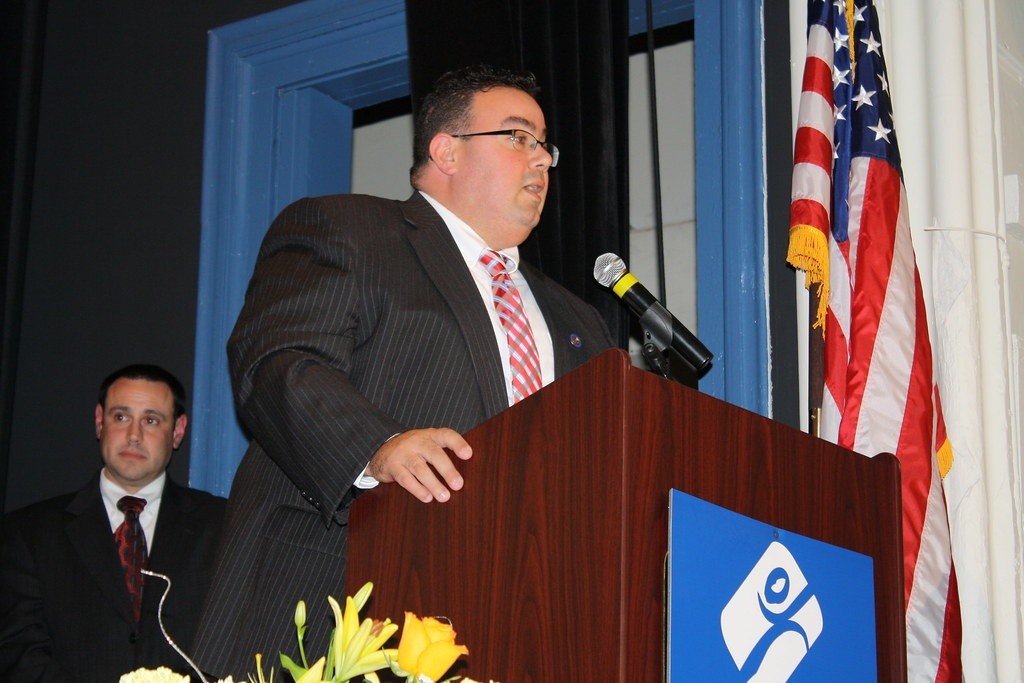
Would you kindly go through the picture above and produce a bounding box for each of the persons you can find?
[183,64,620,683]
[0,364,227,683]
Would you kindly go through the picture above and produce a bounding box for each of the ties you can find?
[111,495,148,626]
[481,250,542,406]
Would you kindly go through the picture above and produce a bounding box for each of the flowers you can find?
[119,581,501,683]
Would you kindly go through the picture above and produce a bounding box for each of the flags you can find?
[786,0,966,683]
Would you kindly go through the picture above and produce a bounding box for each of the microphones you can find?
[594,252,715,371]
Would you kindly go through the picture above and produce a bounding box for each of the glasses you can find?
[428,129,560,168]
